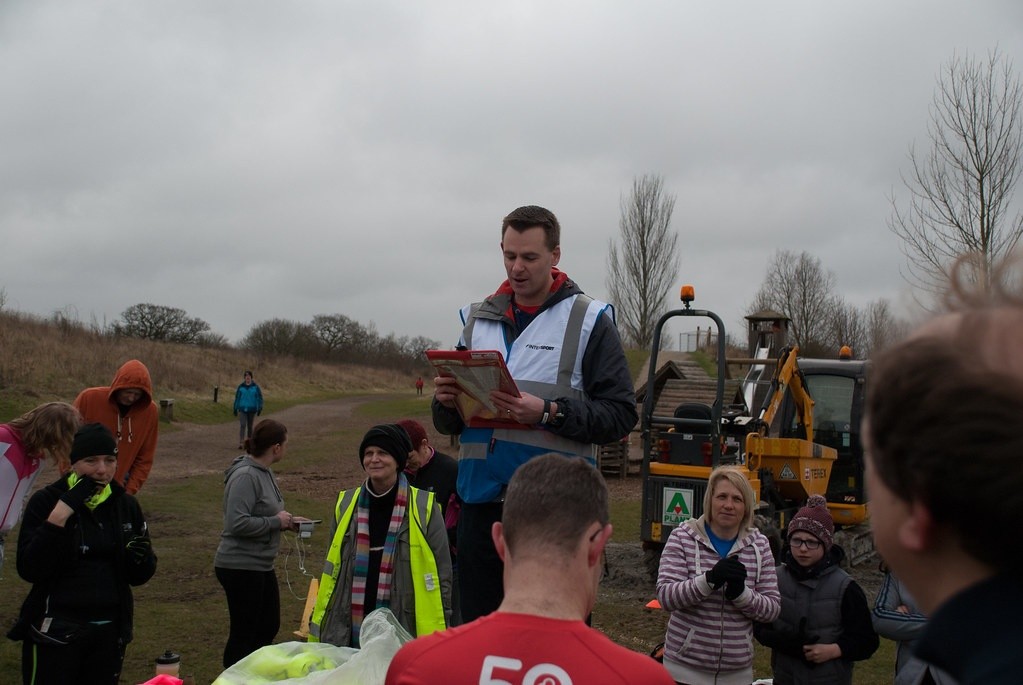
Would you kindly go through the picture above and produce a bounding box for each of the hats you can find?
[360,424,411,474]
[70,423,118,464]
[787,494,834,554]
[243,370,253,379]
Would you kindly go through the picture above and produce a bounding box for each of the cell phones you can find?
[292,519,322,525]
[140,522,147,537]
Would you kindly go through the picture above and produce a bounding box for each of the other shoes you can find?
[238,440,244,449]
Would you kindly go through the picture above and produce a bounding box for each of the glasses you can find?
[789,537,824,549]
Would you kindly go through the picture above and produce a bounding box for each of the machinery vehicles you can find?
[640,284,878,586]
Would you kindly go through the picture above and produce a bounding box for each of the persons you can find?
[6,421,157,685]
[754,496,880,685]
[213,419,311,671]
[432,205,640,628]
[0,402,83,555]
[861,256,1023,685]
[59,359,159,497]
[399,419,462,557]
[655,466,782,685]
[385,454,674,684]
[416,377,424,395]
[307,423,453,652]
[872,570,957,685]
[234,371,264,449]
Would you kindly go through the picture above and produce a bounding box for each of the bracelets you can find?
[536,399,551,426]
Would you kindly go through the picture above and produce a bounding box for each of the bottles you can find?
[155,649,180,680]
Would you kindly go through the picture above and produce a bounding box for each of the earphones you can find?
[299,562,304,570]
[302,567,306,574]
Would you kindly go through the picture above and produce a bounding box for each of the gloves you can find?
[125,527,154,567]
[59,474,105,512]
[706,555,747,601]
[770,617,821,661]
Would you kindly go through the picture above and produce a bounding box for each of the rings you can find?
[508,409,510,415]
